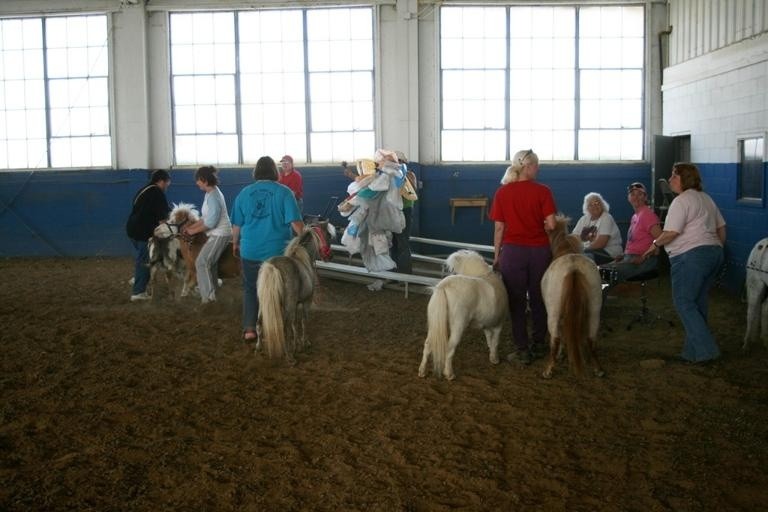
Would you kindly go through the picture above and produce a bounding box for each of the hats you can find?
[396,149,408,165]
[627,181,647,194]
[280,155,293,165]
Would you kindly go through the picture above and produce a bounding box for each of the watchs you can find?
[652,238,661,249]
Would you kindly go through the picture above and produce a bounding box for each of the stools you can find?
[598,260,618,316]
[626,246,664,330]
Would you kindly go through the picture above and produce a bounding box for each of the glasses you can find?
[522,149,533,161]
[626,184,648,192]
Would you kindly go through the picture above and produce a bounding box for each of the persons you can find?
[343,150,418,275]
[490,148,555,365]
[277,154,307,215]
[125,169,169,302]
[641,161,727,365]
[229,155,306,343]
[601,183,663,287]
[185,164,233,307]
[571,191,623,266]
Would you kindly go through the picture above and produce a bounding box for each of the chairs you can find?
[658,179,675,226]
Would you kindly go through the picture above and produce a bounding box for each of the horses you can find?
[741,236,768,350]
[256,218,333,367]
[540,212,609,379]
[154,201,243,301]
[417,249,509,381]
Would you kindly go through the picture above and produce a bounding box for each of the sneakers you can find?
[690,355,723,366]
[244,329,257,342]
[507,348,531,365]
[532,343,549,358]
[671,353,691,365]
[128,277,136,287]
[130,291,151,303]
